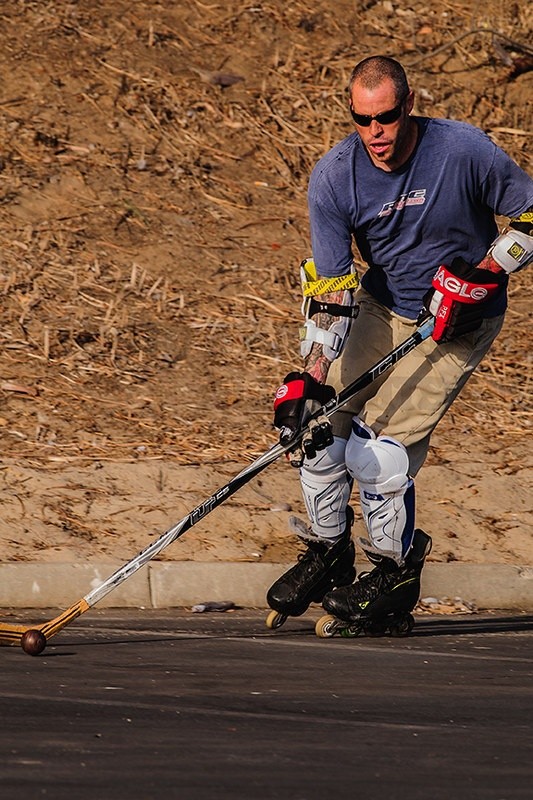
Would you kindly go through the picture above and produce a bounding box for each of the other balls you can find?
[21,629,47,655]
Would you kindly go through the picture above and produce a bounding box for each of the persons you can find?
[259,56,533,639]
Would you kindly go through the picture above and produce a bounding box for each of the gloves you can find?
[271,371,337,469]
[415,258,507,344]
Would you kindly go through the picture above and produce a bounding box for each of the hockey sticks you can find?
[0,311,436,646]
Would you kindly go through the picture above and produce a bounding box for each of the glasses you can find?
[349,101,402,128]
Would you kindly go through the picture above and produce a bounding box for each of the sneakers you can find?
[264,505,358,632]
[314,528,434,638]
